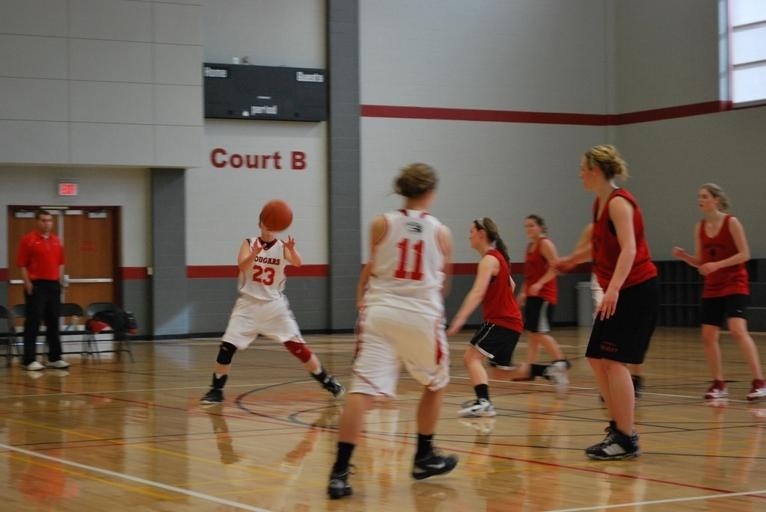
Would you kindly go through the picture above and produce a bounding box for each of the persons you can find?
[199,214,344,404]
[673,183,766,400]
[518,215,563,365]
[446,218,568,417]
[578,224,644,402]
[16,209,71,371]
[551,144,659,461]
[327,163,459,499]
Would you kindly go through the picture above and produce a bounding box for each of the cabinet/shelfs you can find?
[653,260,766,332]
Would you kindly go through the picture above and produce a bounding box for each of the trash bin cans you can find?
[574,281,593,328]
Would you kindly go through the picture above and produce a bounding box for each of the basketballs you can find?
[261,201,293,232]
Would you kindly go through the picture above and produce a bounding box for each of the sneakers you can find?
[322,375,345,397]
[17,361,45,371]
[545,359,571,397]
[585,420,639,461]
[47,361,70,369]
[200,389,224,404]
[329,479,352,498]
[412,453,458,480]
[746,377,766,400]
[704,380,727,398]
[457,395,495,418]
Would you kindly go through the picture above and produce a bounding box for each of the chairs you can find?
[0,303,138,368]
